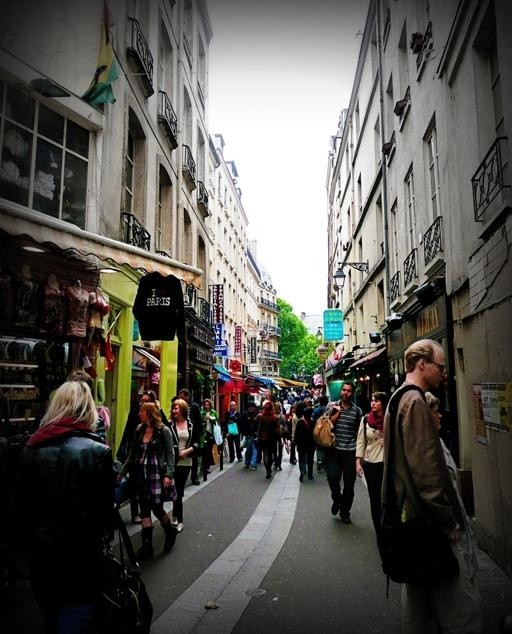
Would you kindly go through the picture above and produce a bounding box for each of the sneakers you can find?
[191,476,200,488]
[328,498,352,525]
[171,513,191,533]
[225,454,323,482]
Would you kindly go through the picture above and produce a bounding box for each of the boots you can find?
[159,519,176,554]
[134,526,154,562]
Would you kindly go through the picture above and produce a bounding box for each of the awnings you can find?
[249,375,309,389]
[350,346,386,369]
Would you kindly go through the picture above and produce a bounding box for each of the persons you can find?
[0,380,154,633]
[117,389,219,554]
[312,381,388,563]
[220,386,315,482]
[383,339,484,634]
[14,266,111,336]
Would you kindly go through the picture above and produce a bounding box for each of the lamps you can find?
[333,259,370,289]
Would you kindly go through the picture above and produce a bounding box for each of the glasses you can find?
[425,360,447,374]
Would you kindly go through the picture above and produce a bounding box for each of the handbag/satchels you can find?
[86,553,157,633]
[377,504,461,586]
